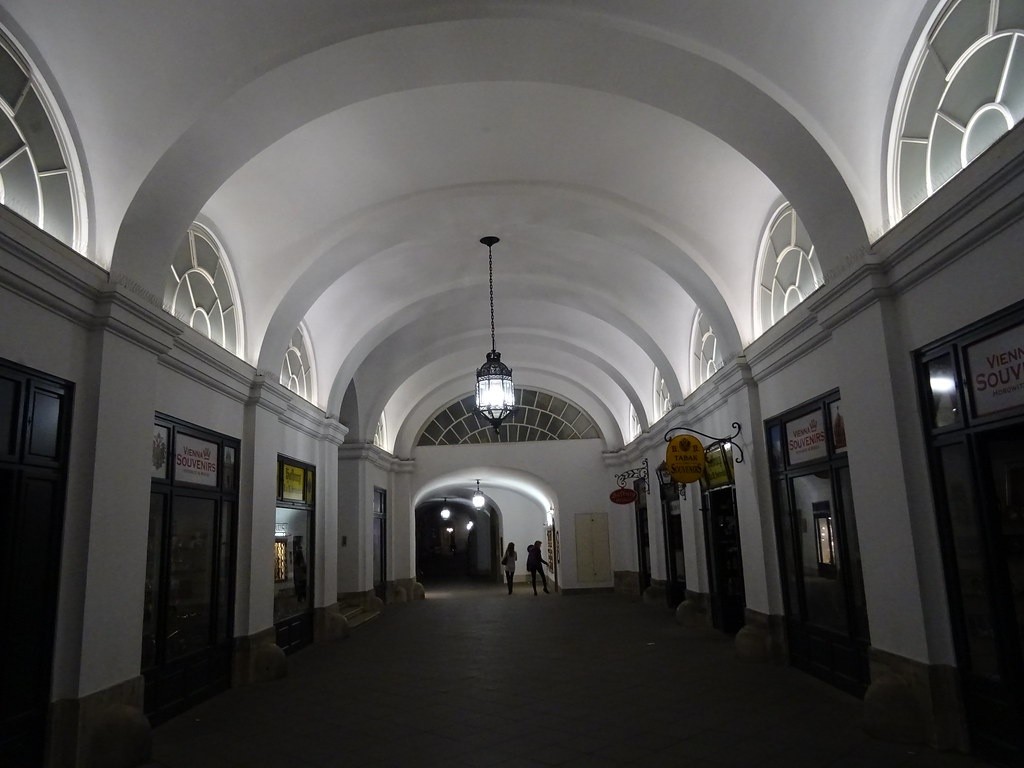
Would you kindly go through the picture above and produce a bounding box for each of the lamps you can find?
[440,496,451,520]
[475,236,520,437]
[471,479,486,510]
[655,460,687,502]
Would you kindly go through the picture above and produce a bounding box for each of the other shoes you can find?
[543,588,549,594]
[534,592,537,595]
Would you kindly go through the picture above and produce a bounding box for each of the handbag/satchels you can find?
[502,551,508,565]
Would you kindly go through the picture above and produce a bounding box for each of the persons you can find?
[502,541,517,595]
[527,541,550,596]
